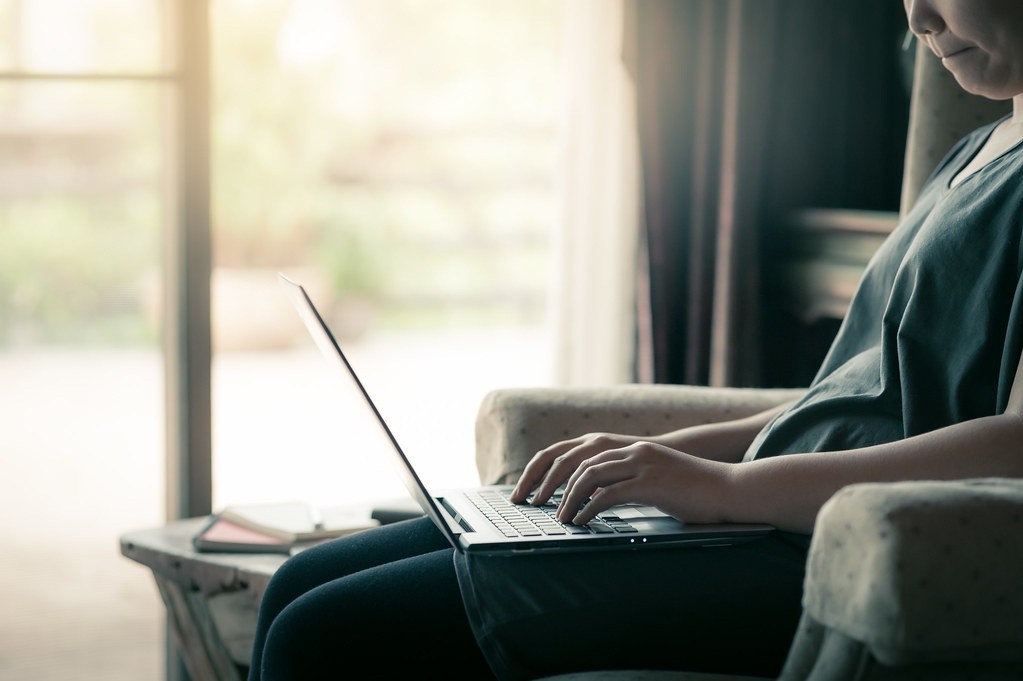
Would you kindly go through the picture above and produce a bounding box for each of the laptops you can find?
[273,272,778,556]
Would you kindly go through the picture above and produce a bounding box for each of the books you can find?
[193,498,381,554]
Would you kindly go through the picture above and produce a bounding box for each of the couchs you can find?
[475,383,1023,681]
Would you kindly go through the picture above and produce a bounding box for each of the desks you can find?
[121,514,358,680]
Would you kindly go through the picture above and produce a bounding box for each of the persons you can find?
[253,0,1023,681]
